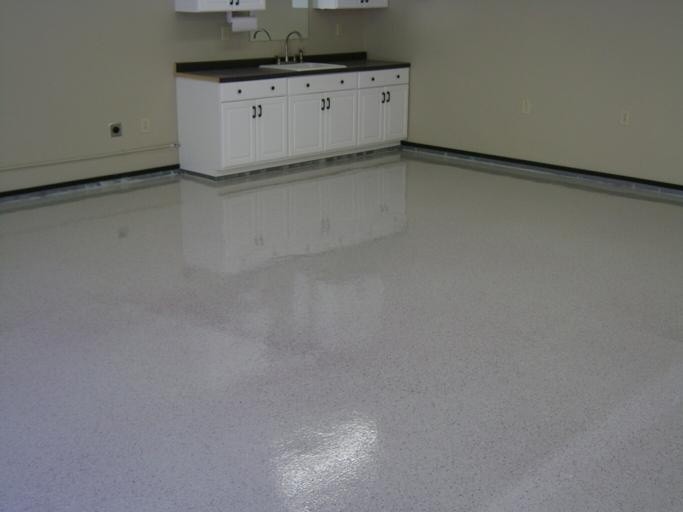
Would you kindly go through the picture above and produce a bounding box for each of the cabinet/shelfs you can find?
[175,77,288,181]
[358,66,410,153]
[313,0,390,10]
[292,0,308,8]
[288,71,358,166]
[174,0,267,13]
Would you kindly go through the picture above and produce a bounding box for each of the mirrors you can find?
[249,0,310,41]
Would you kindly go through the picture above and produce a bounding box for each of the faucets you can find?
[254,29,271,41]
[285,31,302,63]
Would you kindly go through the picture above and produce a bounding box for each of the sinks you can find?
[275,63,338,71]
